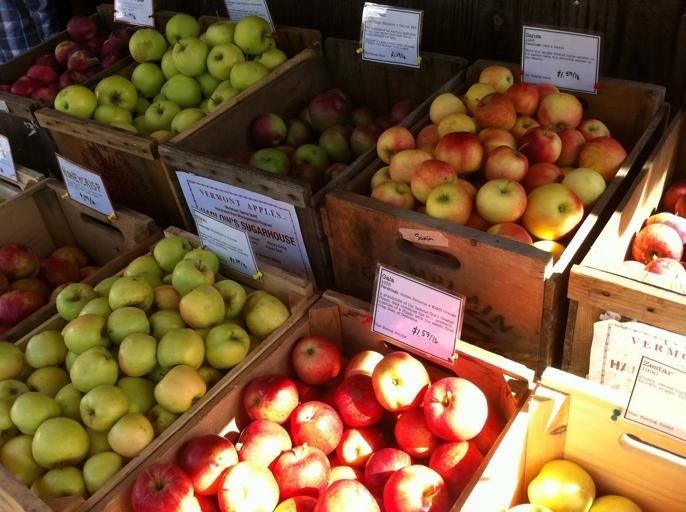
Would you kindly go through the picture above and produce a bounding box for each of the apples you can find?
[0,16,131,104]
[0,237,292,500]
[54,10,289,140]
[507,457,643,512]
[622,177,686,280]
[370,65,627,263]
[128,334,508,512]
[2,240,100,333]
[221,88,422,183]
